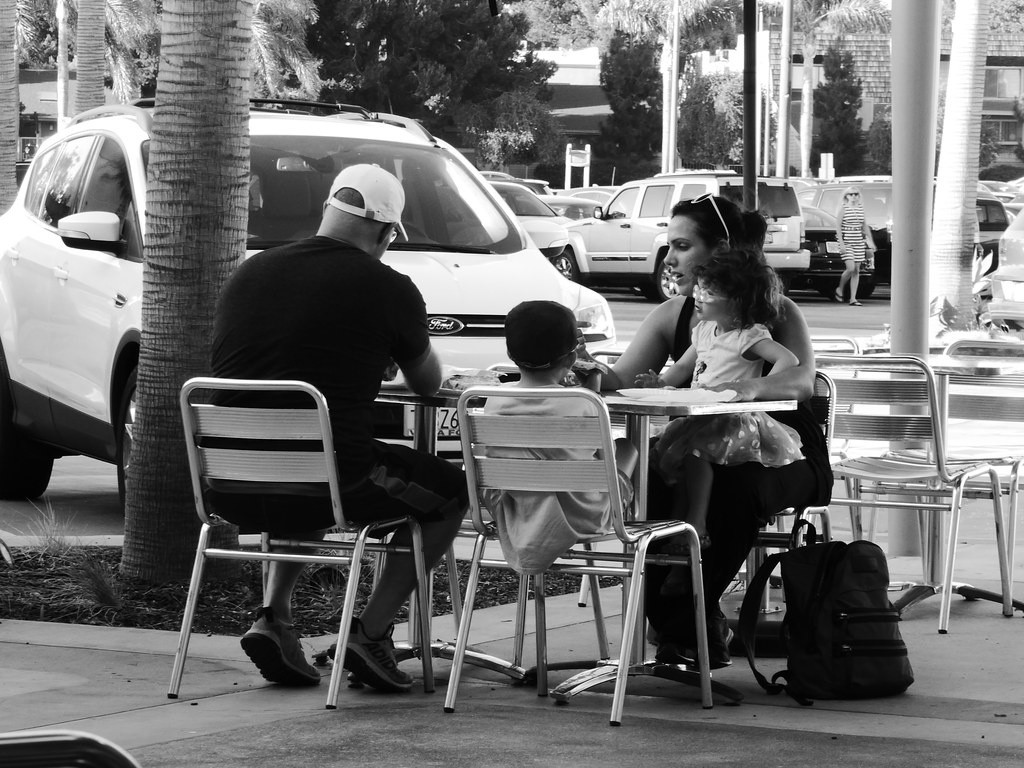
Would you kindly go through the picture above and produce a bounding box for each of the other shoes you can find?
[659,534,711,555]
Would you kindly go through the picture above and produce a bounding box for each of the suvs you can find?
[474,169,1024,330]
[0,98,617,522]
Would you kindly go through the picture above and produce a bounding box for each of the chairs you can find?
[165,337,1024,725]
[256,170,323,239]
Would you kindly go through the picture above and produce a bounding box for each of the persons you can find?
[476,299,640,571]
[195,164,469,693]
[576,193,834,667]
[832,187,876,304]
[632,248,800,554]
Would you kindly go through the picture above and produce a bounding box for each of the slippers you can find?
[849,301,863,306]
[834,286,843,302]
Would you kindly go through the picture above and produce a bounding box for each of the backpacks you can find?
[738,520,916,705]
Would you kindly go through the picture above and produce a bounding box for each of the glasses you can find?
[692,192,732,240]
[692,284,726,303]
[846,193,859,196]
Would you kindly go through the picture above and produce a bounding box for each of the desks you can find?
[374,382,802,706]
[820,351,1024,621]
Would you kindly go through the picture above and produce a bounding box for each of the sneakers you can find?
[655,624,734,667]
[240,606,322,687]
[329,617,414,694]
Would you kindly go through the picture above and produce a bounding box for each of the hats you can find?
[326,164,409,243]
